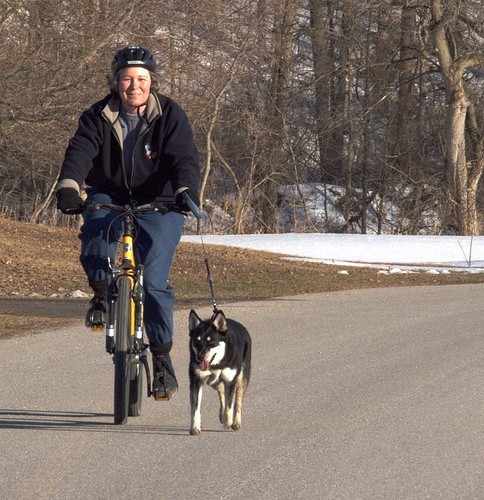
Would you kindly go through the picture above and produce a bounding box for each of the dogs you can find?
[189,309,251,435]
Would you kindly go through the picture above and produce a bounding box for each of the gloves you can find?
[172,186,200,212]
[55,179,84,214]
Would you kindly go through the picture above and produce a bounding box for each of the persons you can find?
[56,47,200,398]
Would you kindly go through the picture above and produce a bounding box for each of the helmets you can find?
[111,45,156,77]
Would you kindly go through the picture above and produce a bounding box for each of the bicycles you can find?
[61,198,188,427]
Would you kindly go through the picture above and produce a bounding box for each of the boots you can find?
[149,340,179,398]
[85,278,108,327]
